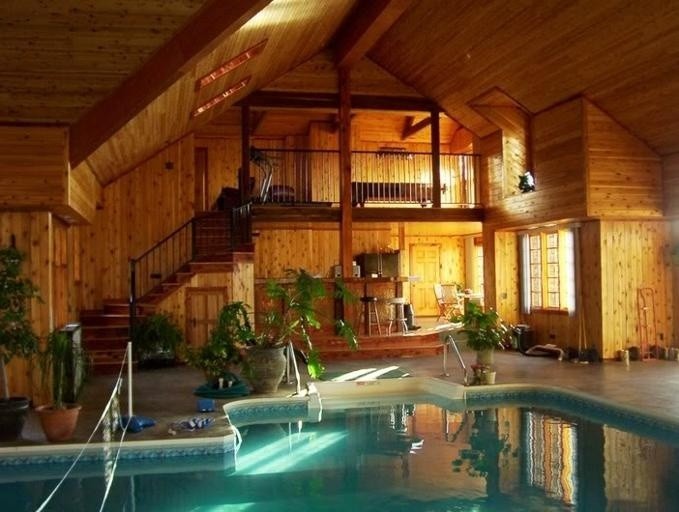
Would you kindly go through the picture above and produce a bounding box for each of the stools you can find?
[356,296,382,338]
[385,297,410,337]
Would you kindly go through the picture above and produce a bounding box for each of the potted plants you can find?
[1,245,47,443]
[195,303,251,401]
[237,271,358,397]
[472,364,496,385]
[33,326,90,440]
[449,301,509,366]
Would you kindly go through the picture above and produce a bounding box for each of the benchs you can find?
[352,182,430,208]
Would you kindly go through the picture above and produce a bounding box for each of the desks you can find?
[456,293,484,316]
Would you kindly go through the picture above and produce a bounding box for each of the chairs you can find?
[432,283,460,322]
